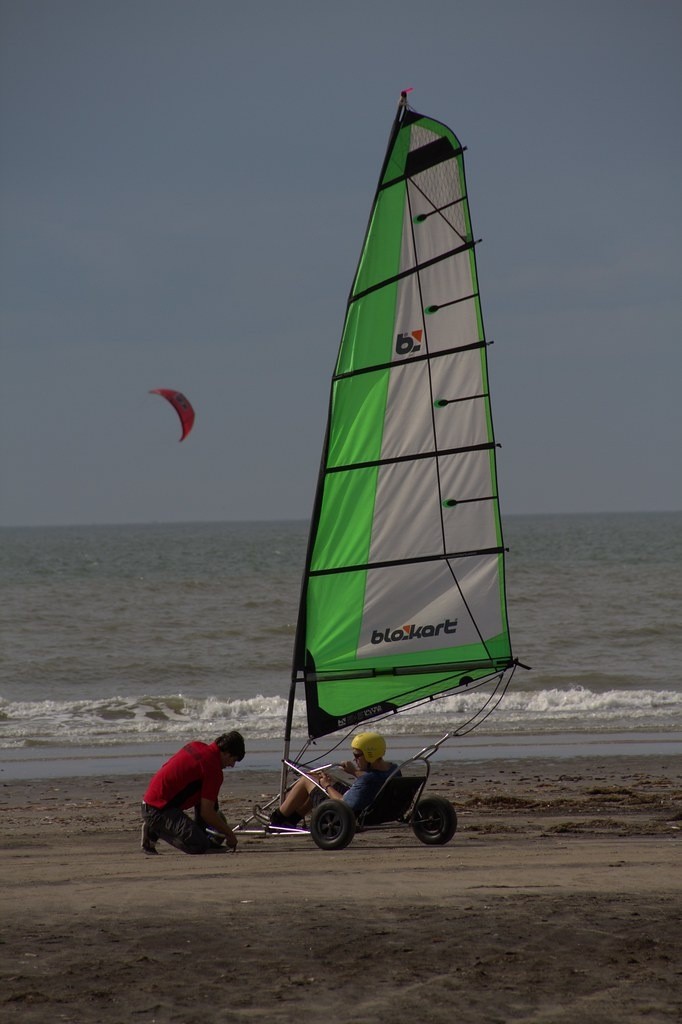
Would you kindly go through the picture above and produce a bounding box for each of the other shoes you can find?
[140,821,160,856]
[264,822,297,833]
[253,804,288,833]
[212,846,227,853]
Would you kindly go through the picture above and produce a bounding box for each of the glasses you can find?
[352,752,363,759]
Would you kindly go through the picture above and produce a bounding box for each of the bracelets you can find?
[325,785,330,794]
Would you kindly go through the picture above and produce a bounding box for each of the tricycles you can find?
[224,760,526,847]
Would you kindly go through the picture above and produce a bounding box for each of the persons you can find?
[140,730,246,855]
[254,732,402,833]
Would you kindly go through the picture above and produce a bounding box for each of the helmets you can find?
[351,731,386,762]
